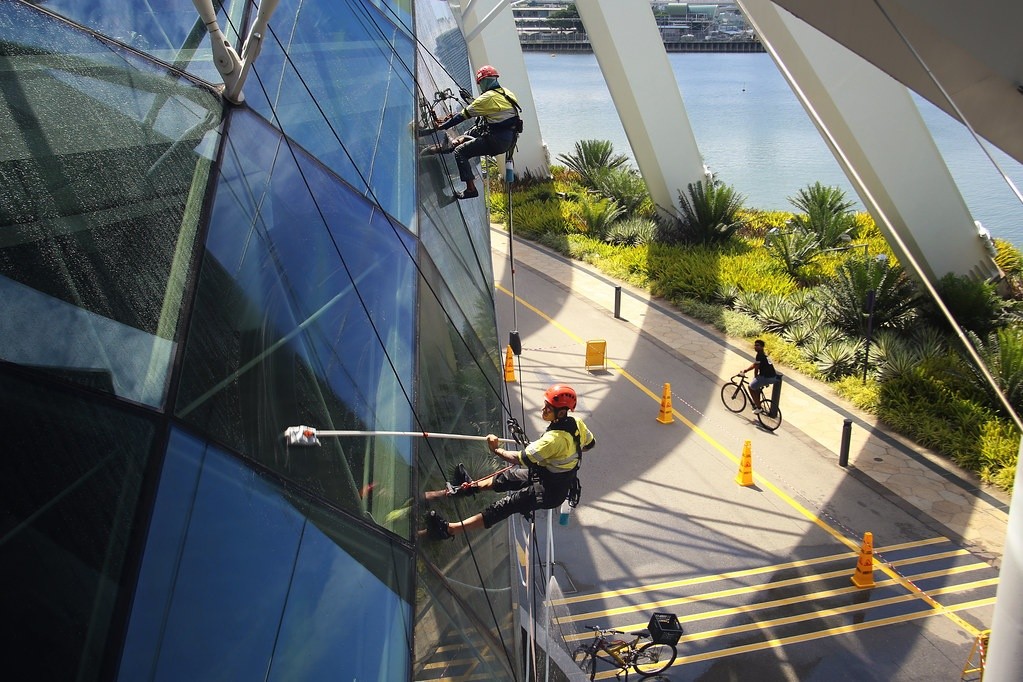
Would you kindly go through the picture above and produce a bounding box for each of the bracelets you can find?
[743,369,747,373]
[494,446,500,454]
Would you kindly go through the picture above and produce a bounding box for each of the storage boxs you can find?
[647,612,684,646]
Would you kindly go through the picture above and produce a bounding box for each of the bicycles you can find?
[720,370,782,432]
[571,624,678,682]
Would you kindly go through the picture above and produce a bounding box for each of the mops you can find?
[282,425,530,447]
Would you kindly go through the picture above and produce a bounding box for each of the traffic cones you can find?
[850,532,878,588]
[655,380,677,425]
[502,343,516,383]
[736,440,757,487]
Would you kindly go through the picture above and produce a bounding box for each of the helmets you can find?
[477,65,500,84]
[543,384,577,412]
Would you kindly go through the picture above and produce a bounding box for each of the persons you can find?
[434,64,523,200]
[740,340,778,414]
[427,384,597,542]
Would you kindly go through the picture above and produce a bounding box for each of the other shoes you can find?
[430,510,456,542]
[446,131,457,151]
[752,408,762,414]
[458,463,475,497]
[456,190,479,199]
